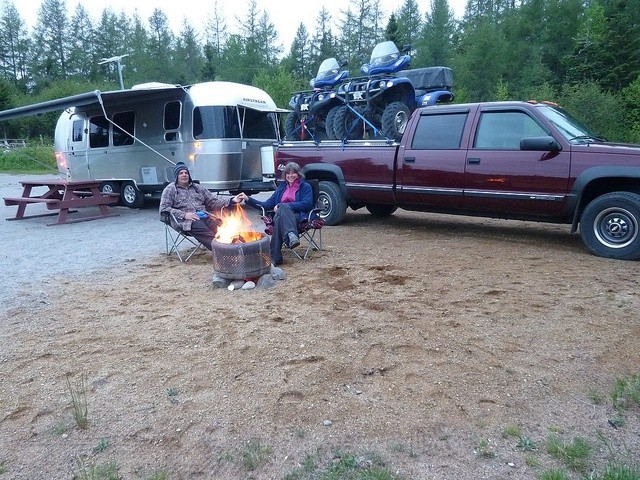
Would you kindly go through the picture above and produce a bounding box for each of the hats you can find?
[173,160,198,194]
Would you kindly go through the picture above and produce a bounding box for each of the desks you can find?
[5,181,120,227]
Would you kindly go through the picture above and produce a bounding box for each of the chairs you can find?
[256,179,322,259]
[162,180,205,261]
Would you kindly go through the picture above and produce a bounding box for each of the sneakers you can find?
[272,258,283,266]
[288,232,300,249]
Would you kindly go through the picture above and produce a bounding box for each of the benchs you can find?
[61,189,121,214]
[3,195,62,226]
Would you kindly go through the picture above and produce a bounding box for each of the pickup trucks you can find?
[275,100,640,259]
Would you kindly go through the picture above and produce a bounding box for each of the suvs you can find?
[333,41,454,140]
[286,57,349,141]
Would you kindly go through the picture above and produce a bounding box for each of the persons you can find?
[237,162,316,267]
[159,161,243,252]
